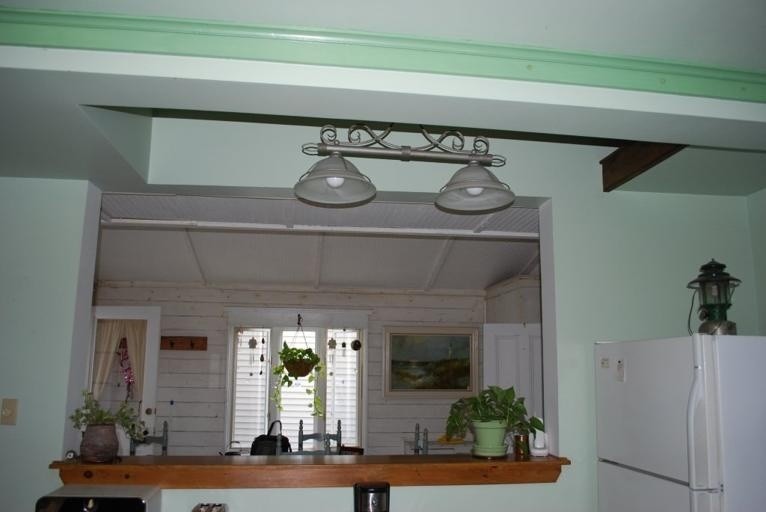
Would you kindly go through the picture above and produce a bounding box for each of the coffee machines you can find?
[352,481,389,512]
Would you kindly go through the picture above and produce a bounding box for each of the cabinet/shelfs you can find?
[482,323,545,456]
[403,437,479,457]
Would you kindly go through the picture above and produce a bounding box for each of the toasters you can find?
[191,502,229,512]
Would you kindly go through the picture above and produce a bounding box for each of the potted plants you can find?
[444,385,545,457]
[67,390,147,465]
[268,340,325,420]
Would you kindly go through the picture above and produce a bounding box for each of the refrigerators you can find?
[592,337,765,512]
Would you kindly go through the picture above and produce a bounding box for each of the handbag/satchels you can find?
[252,435,288,455]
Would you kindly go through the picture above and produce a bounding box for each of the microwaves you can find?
[34,483,161,512]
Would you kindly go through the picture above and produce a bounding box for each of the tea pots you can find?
[217,440,242,456]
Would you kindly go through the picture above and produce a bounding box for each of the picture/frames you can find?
[382,325,480,401]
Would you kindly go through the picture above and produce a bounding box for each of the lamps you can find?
[292,121,515,212]
[684,257,743,336]
